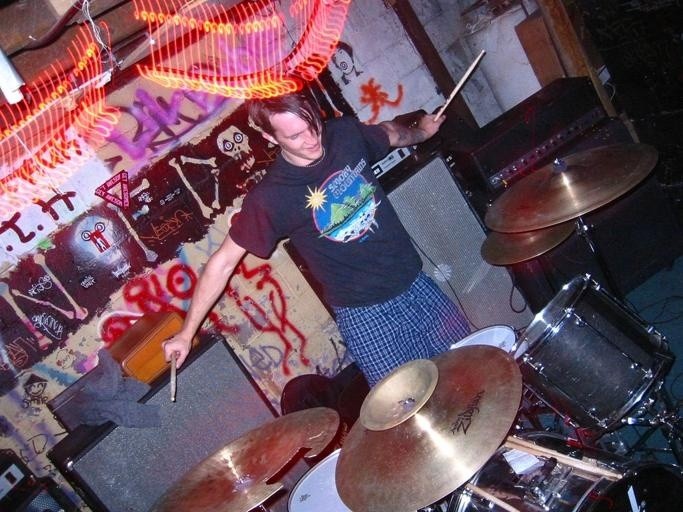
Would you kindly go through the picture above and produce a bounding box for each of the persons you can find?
[161,85,471,391]
[330,41,363,85]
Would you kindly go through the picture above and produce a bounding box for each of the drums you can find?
[450,432,681,511]
[285,448,350,511]
[510,276,670,436]
[450,326,517,353]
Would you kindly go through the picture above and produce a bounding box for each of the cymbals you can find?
[484,144,658,232]
[147,406,339,512]
[335,346,522,511]
[481,221,576,265]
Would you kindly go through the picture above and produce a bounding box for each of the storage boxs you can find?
[46,368,143,432]
[108,310,202,387]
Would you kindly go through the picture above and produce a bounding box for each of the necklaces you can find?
[281,145,326,168]
